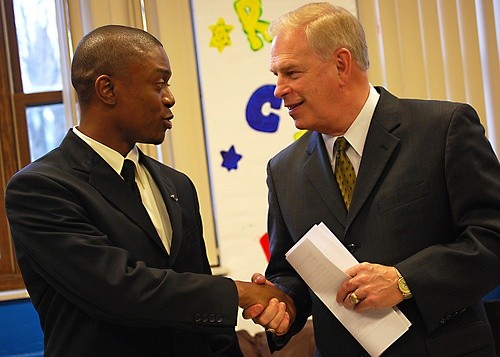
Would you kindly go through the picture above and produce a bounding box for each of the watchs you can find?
[396,268,413,299]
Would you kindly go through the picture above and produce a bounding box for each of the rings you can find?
[266,328,276,333]
[350,292,360,305]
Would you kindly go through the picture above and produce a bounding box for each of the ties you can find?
[121,159,142,202]
[334,137,356,209]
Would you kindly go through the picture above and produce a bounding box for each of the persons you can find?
[242,2,500,357]
[5,25,296,357]
